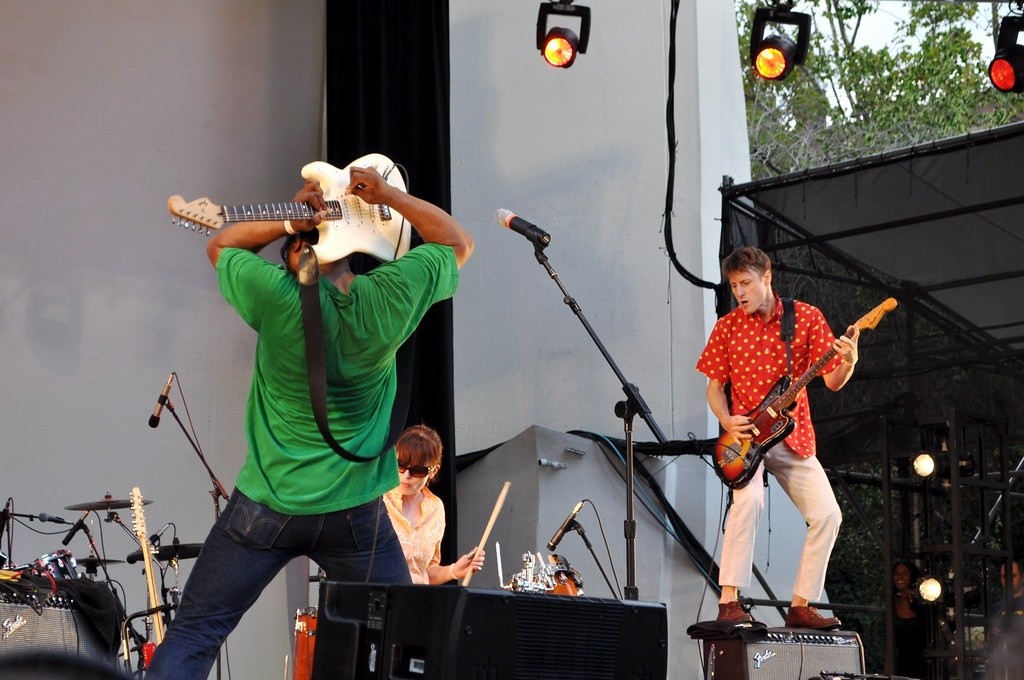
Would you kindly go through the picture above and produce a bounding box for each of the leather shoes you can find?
[785,605,842,630]
[716,601,752,623]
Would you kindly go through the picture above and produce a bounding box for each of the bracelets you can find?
[283,219,297,235]
[449,563,459,580]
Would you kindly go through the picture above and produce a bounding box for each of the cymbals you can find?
[75,556,125,566]
[64,499,154,511]
[129,542,204,561]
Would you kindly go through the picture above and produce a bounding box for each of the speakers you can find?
[702,626,866,680]
[311,582,668,680]
[0,590,117,670]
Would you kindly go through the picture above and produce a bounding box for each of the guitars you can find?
[167,153,412,266]
[129,487,166,670]
[293,605,318,680]
[712,298,899,490]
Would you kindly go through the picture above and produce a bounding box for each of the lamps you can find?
[913,572,982,609]
[750,0,811,82]
[988,0,1024,93]
[910,446,973,480]
[536,0,591,69]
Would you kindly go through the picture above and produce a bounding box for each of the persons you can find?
[146,166,473,680]
[694,247,859,631]
[382,425,485,585]
[990,558,1024,680]
[891,559,930,680]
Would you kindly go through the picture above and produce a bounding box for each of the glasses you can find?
[397,462,436,478]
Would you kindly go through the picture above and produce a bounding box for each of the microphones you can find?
[808,676,853,680]
[62,511,90,546]
[0,498,11,537]
[149,373,174,428]
[547,500,585,552]
[493,209,550,244]
[39,513,73,525]
[127,523,171,564]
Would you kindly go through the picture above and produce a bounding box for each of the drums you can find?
[28,549,81,579]
[510,565,580,596]
